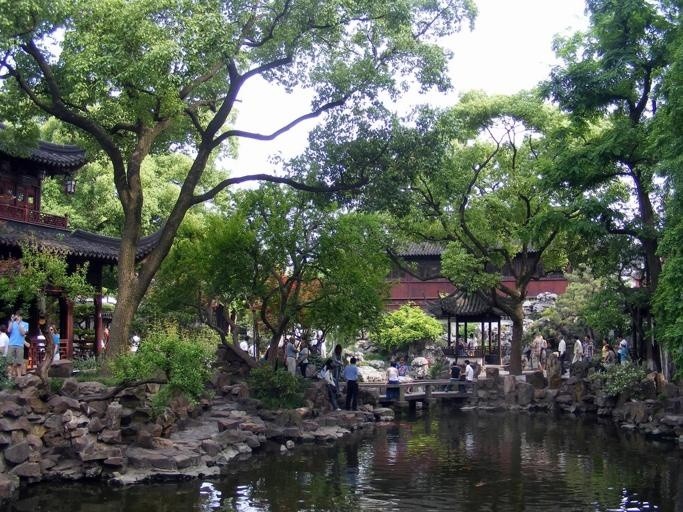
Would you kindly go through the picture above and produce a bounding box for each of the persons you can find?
[5,310,30,382]
[0,309,60,363]
[129,331,139,352]
[466,332,477,356]
[283,334,360,412]
[526,329,630,377]
[248,341,256,360]
[443,362,462,391]
[384,362,399,400]
[457,360,473,393]
[456,334,466,353]
[239,335,248,353]
[396,356,413,393]
